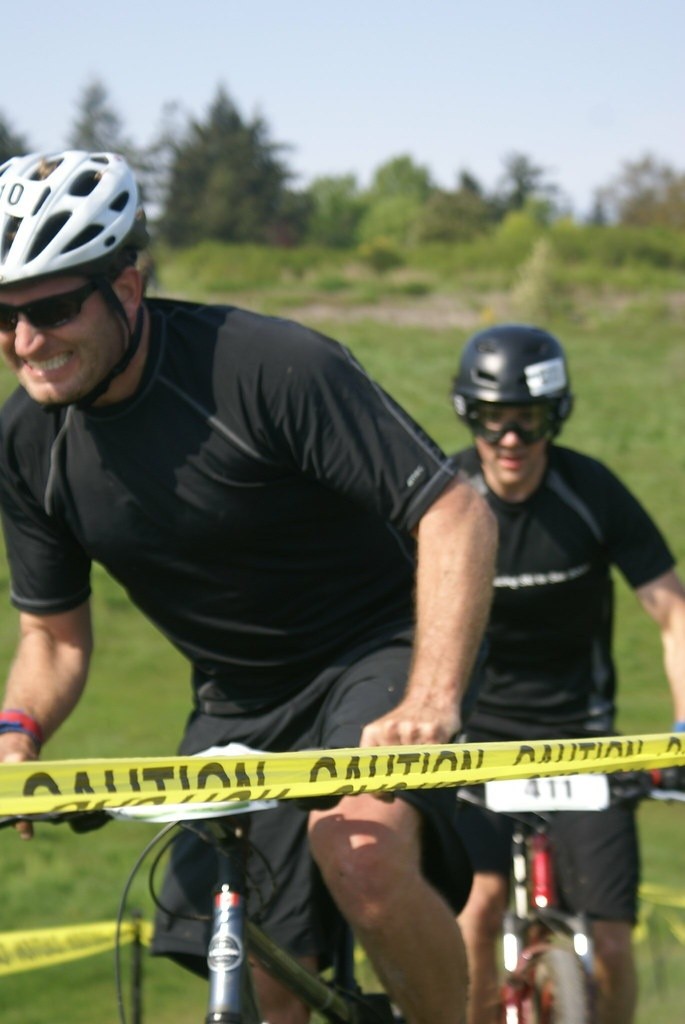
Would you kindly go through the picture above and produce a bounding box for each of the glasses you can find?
[0,256,137,332]
[471,404,554,445]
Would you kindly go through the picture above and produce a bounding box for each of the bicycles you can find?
[455,764,684,1023]
[1,740,404,1024]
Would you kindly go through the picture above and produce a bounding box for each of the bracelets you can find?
[672,722,685,732]
[0,709,43,756]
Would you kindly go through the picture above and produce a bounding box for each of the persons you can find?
[0,148,498,1024]
[446,325,685,1024]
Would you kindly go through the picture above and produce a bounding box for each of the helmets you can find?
[0,148,151,291]
[451,325,586,428]
